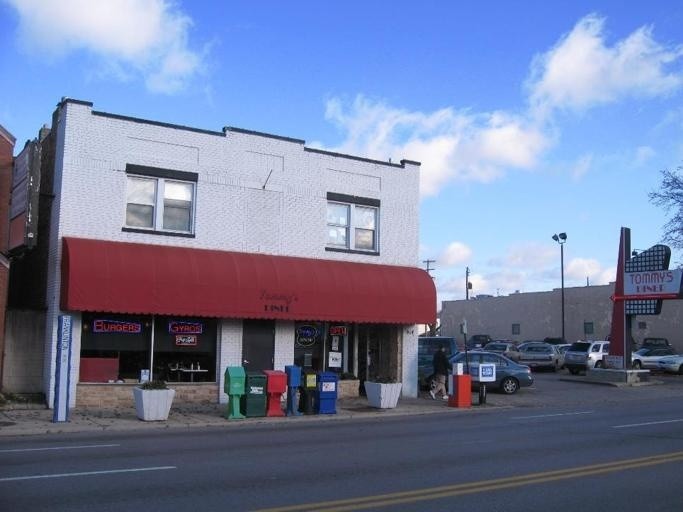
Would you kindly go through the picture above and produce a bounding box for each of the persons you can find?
[429,344,453,400]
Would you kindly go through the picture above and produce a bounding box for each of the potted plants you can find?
[133,380,175,422]
[336,370,403,409]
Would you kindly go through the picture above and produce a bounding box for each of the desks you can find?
[169,368,208,382]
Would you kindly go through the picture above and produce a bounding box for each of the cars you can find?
[418,336,683,394]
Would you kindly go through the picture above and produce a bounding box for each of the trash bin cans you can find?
[447,374,472,408]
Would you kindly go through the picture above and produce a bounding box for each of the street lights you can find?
[553,233,566,339]
